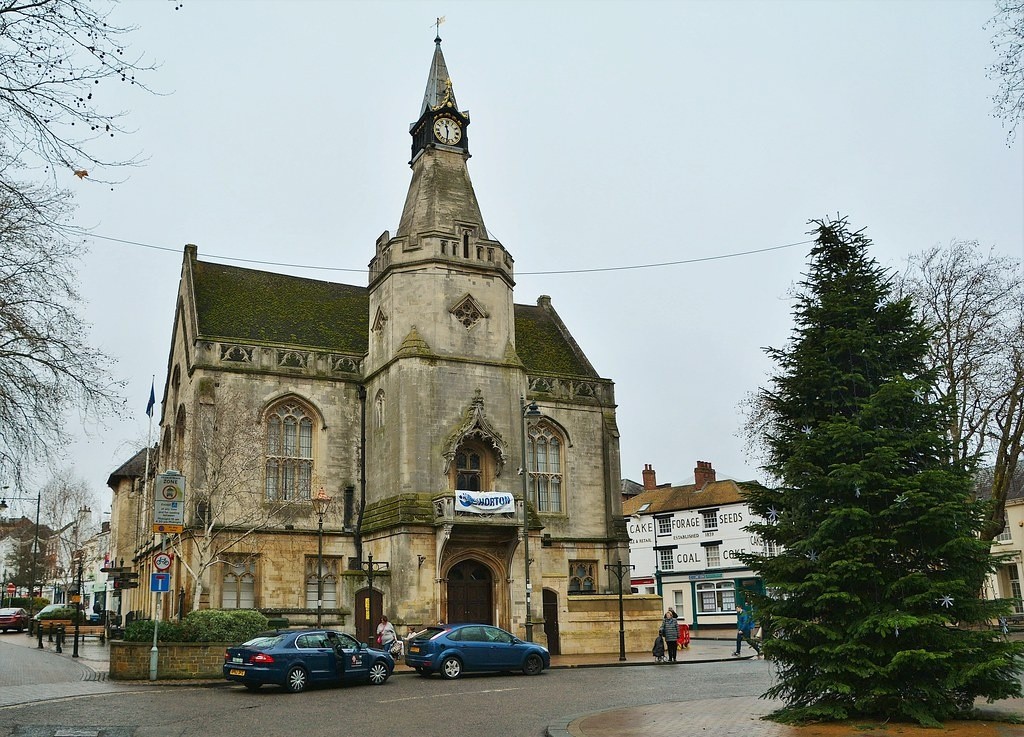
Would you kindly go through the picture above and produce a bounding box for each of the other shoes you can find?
[674,660,676,661]
[734,651,740,655]
[668,658,672,661]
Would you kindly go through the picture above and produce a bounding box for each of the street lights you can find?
[0,490,40,606]
[518,392,545,644]
[312,487,332,629]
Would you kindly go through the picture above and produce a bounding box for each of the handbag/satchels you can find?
[388,641,404,661]
[377,635,382,644]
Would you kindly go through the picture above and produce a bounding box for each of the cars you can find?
[222,628,396,694]
[402,622,551,681]
[33,603,85,622]
[0,607,31,633]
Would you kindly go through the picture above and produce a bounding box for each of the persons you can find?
[93,601,115,624]
[403,627,416,641]
[659,607,678,661]
[732,604,763,656]
[377,615,397,652]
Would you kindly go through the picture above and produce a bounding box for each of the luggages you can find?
[652,636,668,662]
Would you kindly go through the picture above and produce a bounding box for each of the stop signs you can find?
[7,584,15,593]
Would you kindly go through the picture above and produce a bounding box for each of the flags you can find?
[146,382,155,418]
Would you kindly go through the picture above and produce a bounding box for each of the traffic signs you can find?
[100,567,139,589]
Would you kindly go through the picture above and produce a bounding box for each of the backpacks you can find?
[748,614,755,629]
